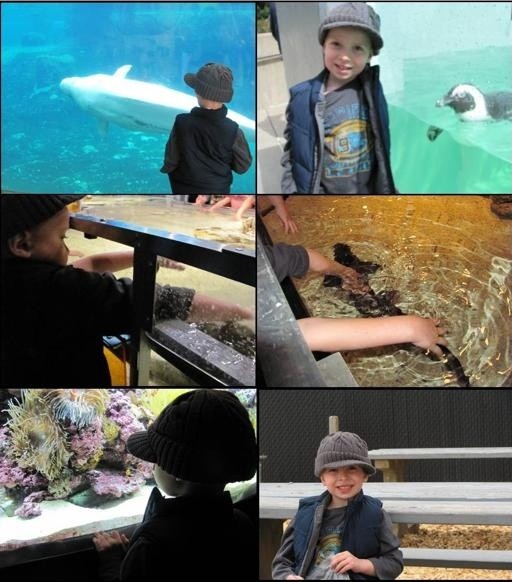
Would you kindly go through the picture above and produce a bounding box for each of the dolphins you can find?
[60,64,255,143]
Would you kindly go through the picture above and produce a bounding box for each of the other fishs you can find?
[323,243,470,387]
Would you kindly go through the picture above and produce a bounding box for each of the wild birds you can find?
[434,83,512,122]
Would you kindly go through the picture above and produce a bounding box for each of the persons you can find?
[91,389,260,581]
[161,62,252,195]
[269,431,403,582]
[201,195,255,221]
[1,193,256,388]
[192,195,209,208]
[279,2,401,194]
[264,241,448,360]
[260,193,301,234]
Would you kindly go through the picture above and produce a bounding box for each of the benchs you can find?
[259,447,512,580]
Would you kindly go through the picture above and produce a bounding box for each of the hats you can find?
[0,194,85,242]
[318,2,383,50]
[128,390,257,483]
[314,432,375,476]
[184,63,233,102]
